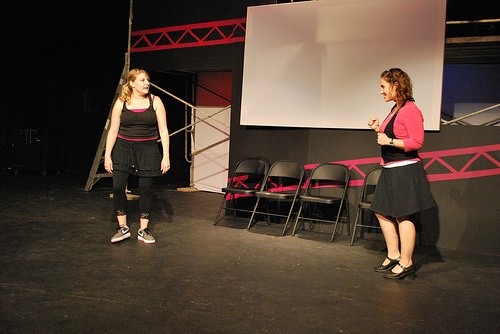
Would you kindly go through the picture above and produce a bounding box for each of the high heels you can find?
[373,256,416,282]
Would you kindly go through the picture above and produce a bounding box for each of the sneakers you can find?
[111,226,156,244]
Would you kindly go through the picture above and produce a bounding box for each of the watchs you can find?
[389,137,395,148]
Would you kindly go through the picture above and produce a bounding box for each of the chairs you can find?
[247,161,304,236]
[350,167,383,246]
[292,163,350,242]
[214,157,268,231]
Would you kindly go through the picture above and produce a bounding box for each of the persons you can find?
[368,67,439,280]
[104,68,169,244]
